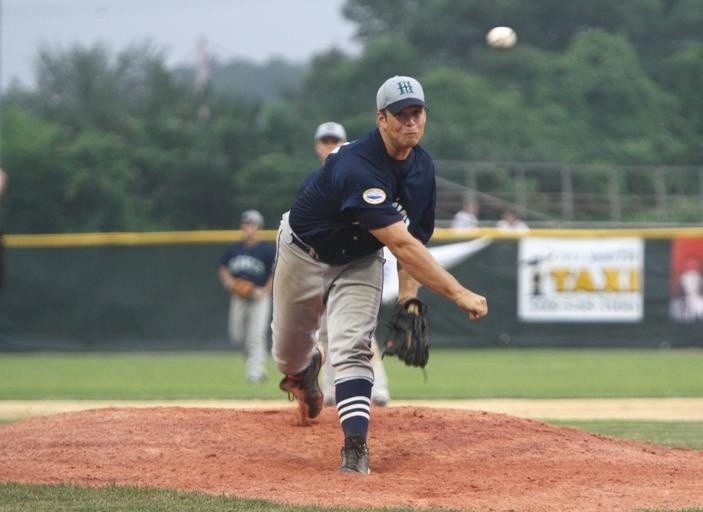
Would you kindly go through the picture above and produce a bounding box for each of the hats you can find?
[376,75,430,115]
[243,210,264,228]
[316,122,345,140]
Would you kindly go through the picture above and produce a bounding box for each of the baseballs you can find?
[485,26,516,48]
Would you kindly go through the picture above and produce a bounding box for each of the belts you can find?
[289,233,325,262]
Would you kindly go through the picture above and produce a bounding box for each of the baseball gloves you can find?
[382,295,431,368]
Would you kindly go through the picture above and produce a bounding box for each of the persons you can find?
[314,120,389,407]
[270,75,488,475]
[498,210,530,232]
[453,197,479,229]
[218,209,277,385]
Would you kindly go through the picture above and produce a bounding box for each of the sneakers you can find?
[336,433,370,476]
[372,389,388,405]
[323,386,337,405]
[280,341,326,418]
